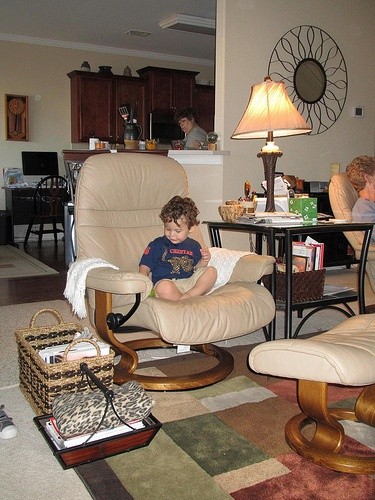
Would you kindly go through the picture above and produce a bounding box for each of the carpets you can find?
[72,329,375,500]
[0,244,59,278]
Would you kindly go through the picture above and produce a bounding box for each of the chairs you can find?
[72,153,276,390]
[328,172,374,292]
[23,175,70,255]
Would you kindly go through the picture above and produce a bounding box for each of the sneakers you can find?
[0,404,17,439]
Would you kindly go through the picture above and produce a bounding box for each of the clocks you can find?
[3,94,28,141]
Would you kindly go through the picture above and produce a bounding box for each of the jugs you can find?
[122,122,143,139]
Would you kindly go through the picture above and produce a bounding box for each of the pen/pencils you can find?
[245,181,257,201]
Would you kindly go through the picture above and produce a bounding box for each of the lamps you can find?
[230,75,312,222]
[159,14,216,37]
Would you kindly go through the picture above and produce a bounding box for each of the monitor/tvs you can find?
[21,151,58,183]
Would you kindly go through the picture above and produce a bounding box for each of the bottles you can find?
[139,139,146,150]
[174,140,185,151]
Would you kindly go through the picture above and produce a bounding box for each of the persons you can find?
[139,196,217,301]
[179,112,208,150]
[348,156,375,242]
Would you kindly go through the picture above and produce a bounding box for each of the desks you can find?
[201,220,374,339]
[308,192,335,220]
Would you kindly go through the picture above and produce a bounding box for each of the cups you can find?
[225,199,240,206]
[239,201,256,216]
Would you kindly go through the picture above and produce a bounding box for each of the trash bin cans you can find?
[0,210,11,245]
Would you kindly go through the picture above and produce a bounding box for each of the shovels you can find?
[119,106,129,122]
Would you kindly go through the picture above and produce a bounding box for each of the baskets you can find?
[263,268,327,303]
[15,309,162,470]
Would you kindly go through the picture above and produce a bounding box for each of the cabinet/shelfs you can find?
[3,187,72,249]
[67,65,215,144]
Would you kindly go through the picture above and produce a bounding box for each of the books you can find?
[39,341,111,364]
[45,418,145,448]
[292,241,324,272]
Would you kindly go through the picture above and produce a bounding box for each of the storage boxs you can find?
[289,197,318,223]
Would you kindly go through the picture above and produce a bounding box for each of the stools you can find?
[248,314,375,474]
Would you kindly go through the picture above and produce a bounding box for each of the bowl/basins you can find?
[218,205,244,222]
[86,137,139,150]
